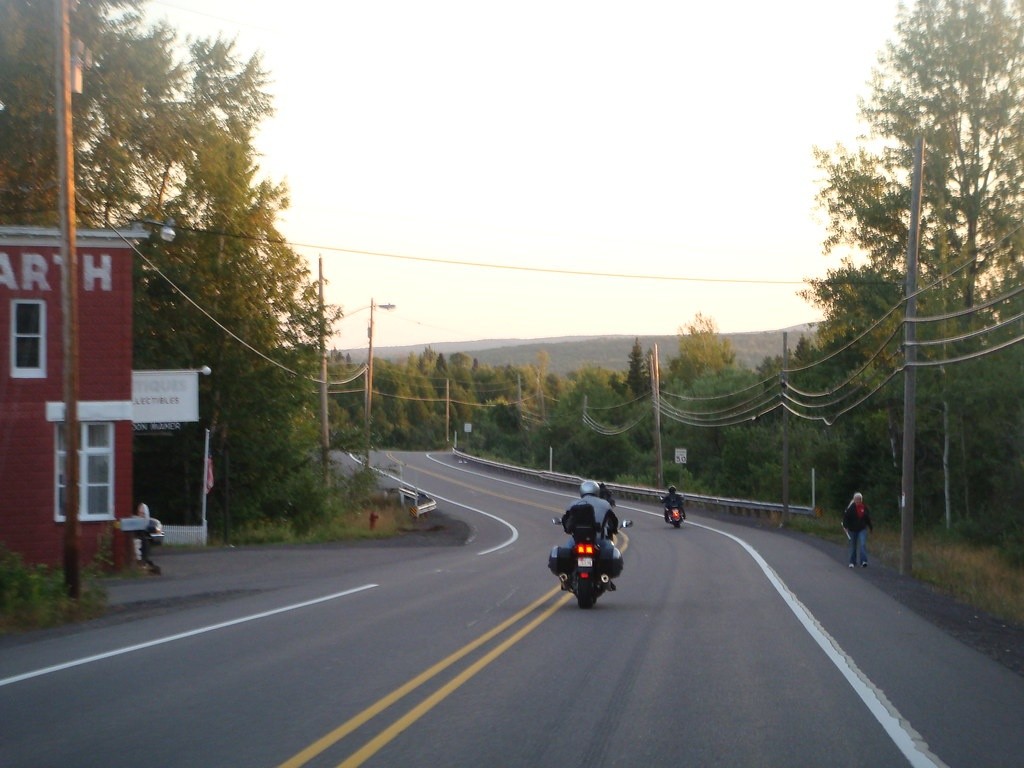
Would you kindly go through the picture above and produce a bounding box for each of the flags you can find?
[206,434,214,494]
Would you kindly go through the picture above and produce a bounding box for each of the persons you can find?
[842,492,874,568]
[562,479,619,549]
[660,485,687,524]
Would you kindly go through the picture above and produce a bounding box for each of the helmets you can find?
[579,481,600,498]
[669,486,676,493]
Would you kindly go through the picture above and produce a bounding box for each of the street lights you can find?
[364,297,396,471]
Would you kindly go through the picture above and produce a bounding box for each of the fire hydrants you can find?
[369,511,379,531]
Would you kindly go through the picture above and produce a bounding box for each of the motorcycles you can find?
[548,518,634,609]
[659,493,688,528]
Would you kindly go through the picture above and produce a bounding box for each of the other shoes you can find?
[610,581,616,591]
[861,562,868,568]
[849,563,854,568]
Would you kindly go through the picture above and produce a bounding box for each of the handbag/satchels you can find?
[567,500,595,533]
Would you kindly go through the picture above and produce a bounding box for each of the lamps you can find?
[199,365,212,375]
[159,225,176,243]
